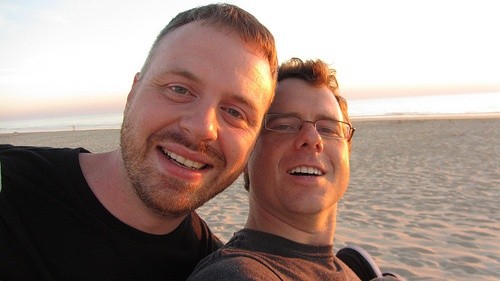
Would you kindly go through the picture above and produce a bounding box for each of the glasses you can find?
[265,112,356,142]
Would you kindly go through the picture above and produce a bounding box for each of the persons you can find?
[187,58,361,281]
[1,3,278,281]
